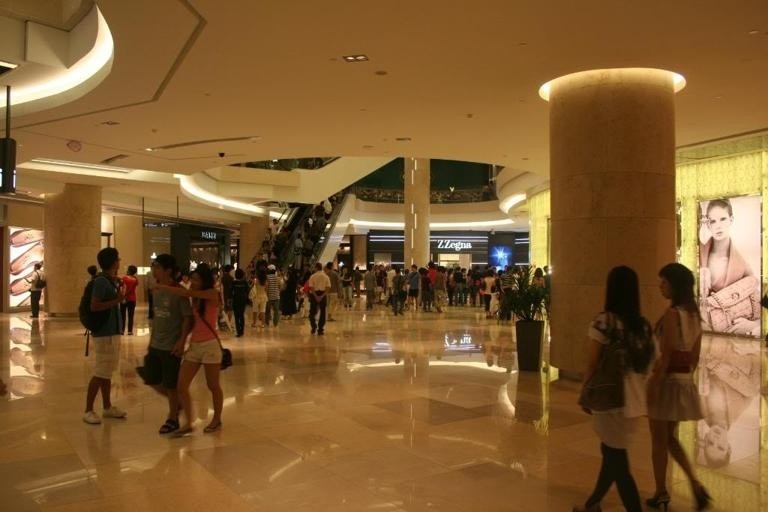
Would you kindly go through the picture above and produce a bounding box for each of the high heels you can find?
[646,492,669,512]
[694,487,710,511]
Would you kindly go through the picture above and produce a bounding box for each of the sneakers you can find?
[574,503,602,512]
[82,407,221,438]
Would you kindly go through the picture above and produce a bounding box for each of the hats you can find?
[267,265,274,270]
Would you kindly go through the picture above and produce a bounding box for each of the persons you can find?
[22,317,49,373]
[646,263,715,512]
[88,259,339,337]
[700,201,761,339]
[571,265,655,512]
[143,437,196,489]
[156,264,223,437]
[697,334,761,471]
[83,247,128,424]
[24,263,45,317]
[252,196,333,276]
[113,257,124,334]
[136,254,193,434]
[190,309,550,383]
[80,424,120,484]
[338,260,551,324]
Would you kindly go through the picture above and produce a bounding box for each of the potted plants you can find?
[495,265,550,370]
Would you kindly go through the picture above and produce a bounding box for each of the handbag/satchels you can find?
[248,287,257,302]
[36,280,45,289]
[580,353,623,412]
[705,276,760,332]
[220,349,231,369]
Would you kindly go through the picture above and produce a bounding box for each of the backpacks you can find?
[79,275,118,330]
